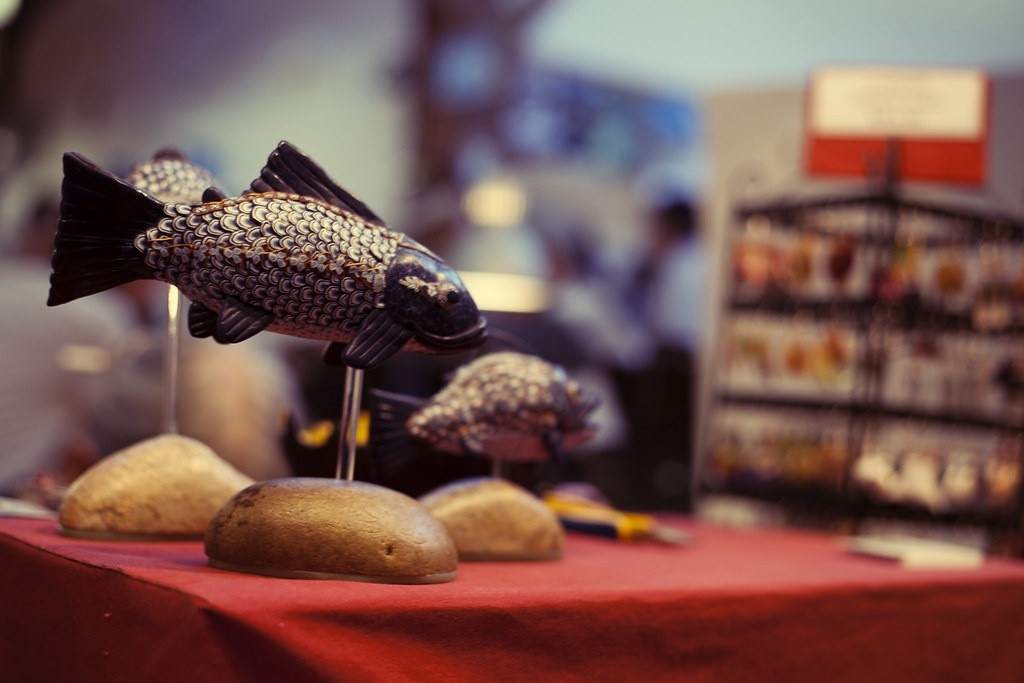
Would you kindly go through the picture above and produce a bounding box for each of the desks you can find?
[0,502,1024,681]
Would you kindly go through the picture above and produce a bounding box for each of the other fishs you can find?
[117,146,230,211]
[369,350,602,468]
[47,141,490,369]
